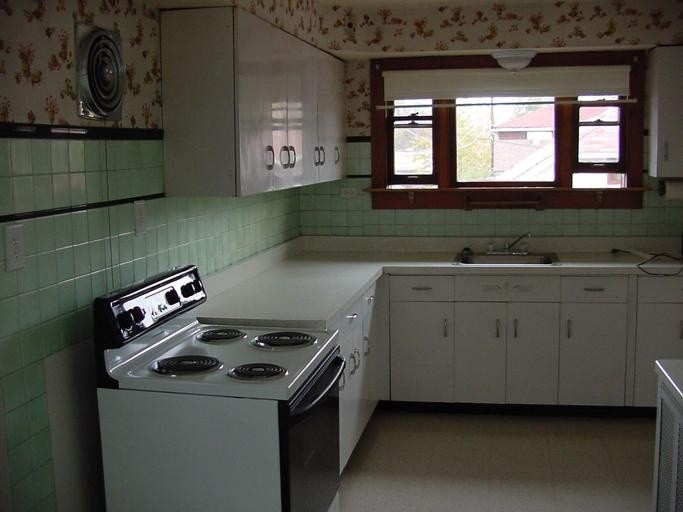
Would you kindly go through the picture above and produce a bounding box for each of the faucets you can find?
[503,231,532,250]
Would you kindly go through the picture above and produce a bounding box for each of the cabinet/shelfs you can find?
[648,44,683,179]
[634,275,683,406]
[389,275,454,403]
[559,275,628,407]
[161,6,346,199]
[337,295,381,477]
[454,274,559,406]
[361,276,385,434]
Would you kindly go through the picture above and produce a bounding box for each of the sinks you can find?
[460,254,554,264]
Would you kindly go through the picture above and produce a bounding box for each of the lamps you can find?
[490,50,538,71]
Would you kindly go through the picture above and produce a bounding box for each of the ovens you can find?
[96,346,348,511]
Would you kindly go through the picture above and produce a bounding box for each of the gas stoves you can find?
[106,317,339,400]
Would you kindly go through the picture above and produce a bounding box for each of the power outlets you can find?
[134,200,146,236]
[5,223,25,272]
